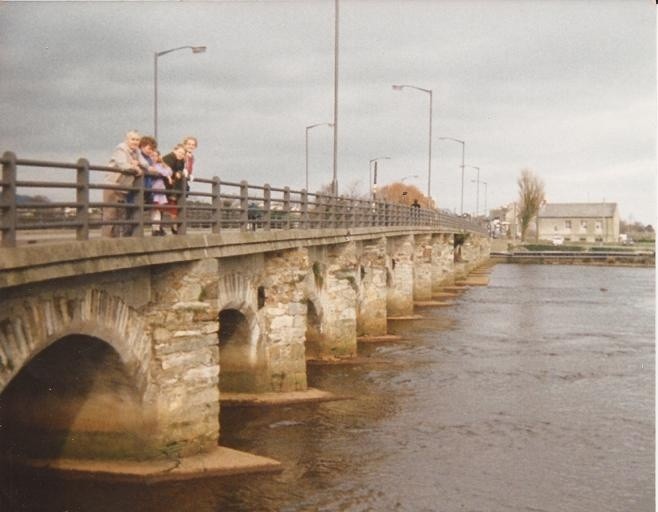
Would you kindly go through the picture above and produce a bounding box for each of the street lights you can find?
[437,136,465,216]
[305,122,334,190]
[458,165,480,213]
[368,157,390,200]
[154,42,207,141]
[392,84,433,206]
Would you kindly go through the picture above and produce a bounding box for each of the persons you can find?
[100,127,197,235]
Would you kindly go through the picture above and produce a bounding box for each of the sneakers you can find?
[151,226,177,236]
